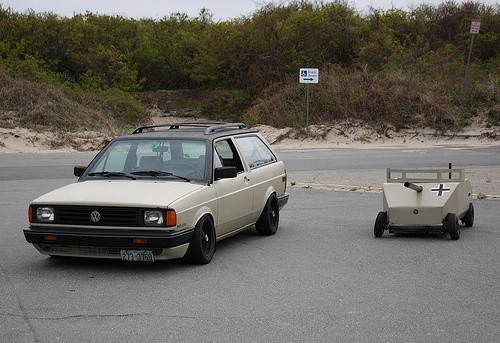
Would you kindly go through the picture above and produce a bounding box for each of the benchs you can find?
[168,159,241,167]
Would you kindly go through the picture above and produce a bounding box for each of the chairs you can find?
[140,156,163,172]
[195,156,206,169]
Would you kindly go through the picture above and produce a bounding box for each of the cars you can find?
[22,121,290,265]
